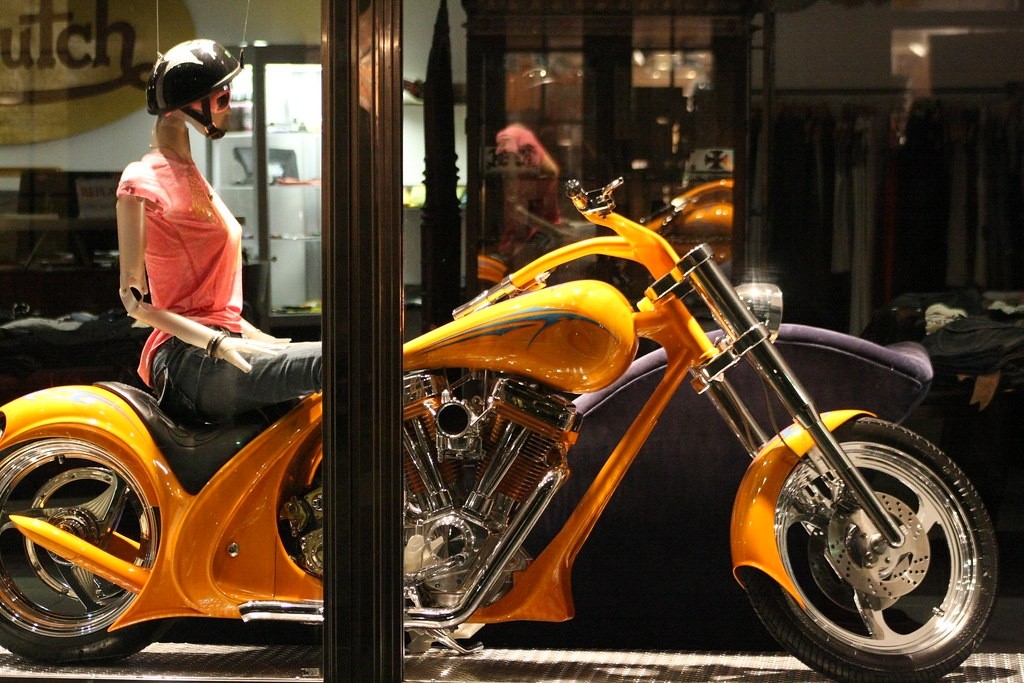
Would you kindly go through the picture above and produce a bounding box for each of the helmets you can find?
[145,38,241,116]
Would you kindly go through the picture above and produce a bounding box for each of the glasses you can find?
[164,89,231,117]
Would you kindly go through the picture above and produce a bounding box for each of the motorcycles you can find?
[0,174,1005,683]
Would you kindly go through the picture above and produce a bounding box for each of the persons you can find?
[116,38,486,654]
[494,66,596,279]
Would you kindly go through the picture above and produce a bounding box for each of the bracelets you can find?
[206,332,225,357]
